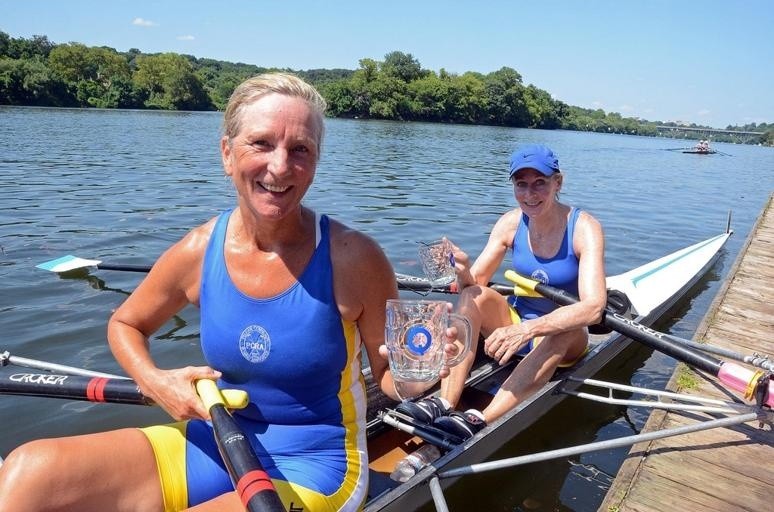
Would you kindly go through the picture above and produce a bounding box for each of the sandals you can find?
[433,412,482,436]
[397,395,448,420]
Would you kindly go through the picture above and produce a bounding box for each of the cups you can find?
[417,239,458,286]
[385,297,472,380]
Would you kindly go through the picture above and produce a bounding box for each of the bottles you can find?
[387,445,439,484]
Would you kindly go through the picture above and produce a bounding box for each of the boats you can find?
[361,209,743,512]
[680,149,718,154]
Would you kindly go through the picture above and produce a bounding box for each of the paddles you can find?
[35,256,547,298]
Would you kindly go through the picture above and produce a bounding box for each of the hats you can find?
[507,145,560,181]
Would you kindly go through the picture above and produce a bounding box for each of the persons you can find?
[0,70,459,511]
[696,140,703,151]
[701,141,710,151]
[394,143,607,438]
